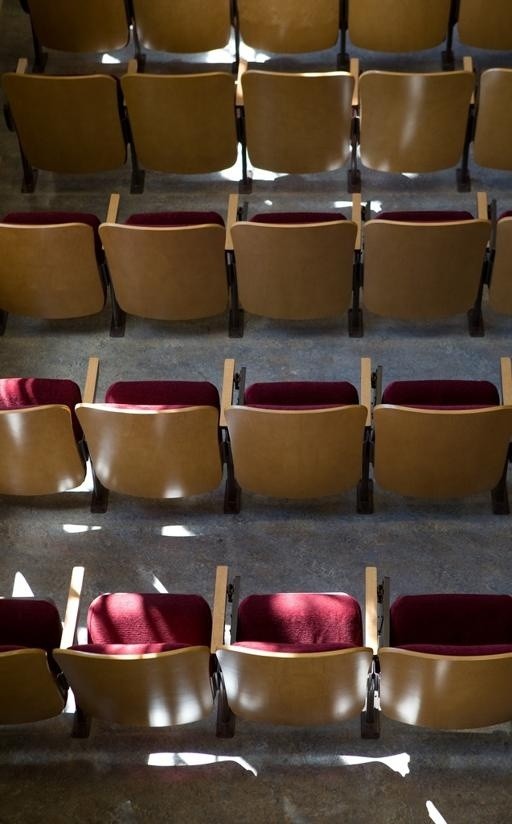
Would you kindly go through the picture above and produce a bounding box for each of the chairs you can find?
[203,561,375,748]
[0,1,511,520]
[355,559,509,745]
[54,563,232,740]
[4,557,78,722]
